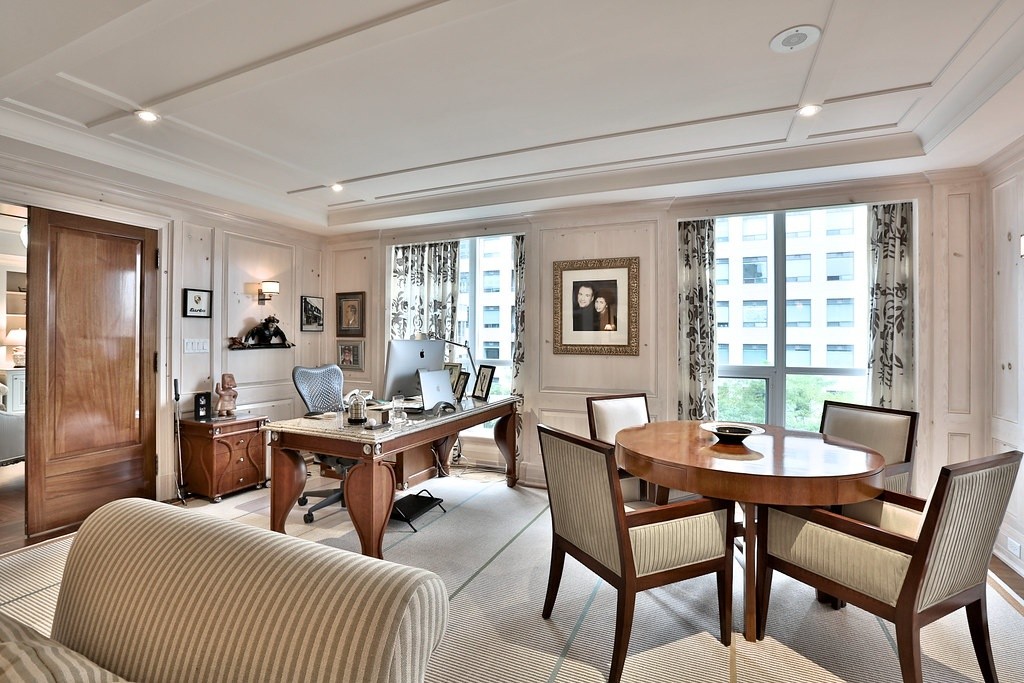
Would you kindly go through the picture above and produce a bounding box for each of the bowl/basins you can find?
[698,422,766,443]
[697,441,764,461]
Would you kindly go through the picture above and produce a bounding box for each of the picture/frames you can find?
[184,288,213,319]
[194,392,211,421]
[471,364,497,402]
[553,257,642,355]
[442,361,462,393]
[300,296,325,333]
[337,340,365,373]
[336,291,366,338]
[453,371,471,402]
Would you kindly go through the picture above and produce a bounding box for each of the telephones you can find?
[343,389,374,405]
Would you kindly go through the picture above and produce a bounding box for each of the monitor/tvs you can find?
[382,340,454,410]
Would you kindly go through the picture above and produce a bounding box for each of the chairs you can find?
[816,399,920,611]
[292,364,361,524]
[535,422,736,683]
[585,391,704,505]
[756,449,1024,683]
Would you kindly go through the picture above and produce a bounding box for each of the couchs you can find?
[0,411,26,467]
[0,497,451,683]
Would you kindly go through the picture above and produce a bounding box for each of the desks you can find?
[615,420,886,646]
[259,393,524,560]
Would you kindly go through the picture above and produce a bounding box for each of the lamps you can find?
[0,327,27,370]
[258,281,280,305]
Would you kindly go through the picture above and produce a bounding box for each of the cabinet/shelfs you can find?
[0,254,28,368]
[320,441,438,492]
[175,413,273,504]
[0,368,27,413]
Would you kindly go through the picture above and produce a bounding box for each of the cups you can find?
[392,395,404,414]
